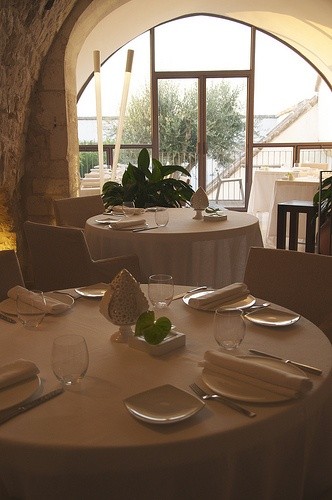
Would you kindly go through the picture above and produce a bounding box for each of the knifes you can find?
[0,388,65,425]
[249,348,322,376]
[132,227,158,232]
[164,285,207,301]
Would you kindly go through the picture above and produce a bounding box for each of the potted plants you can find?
[312,176,332,256]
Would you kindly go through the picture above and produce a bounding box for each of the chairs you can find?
[243,246,332,346]
[0,249,26,303]
[215,168,245,204]
[23,221,137,294]
[53,195,106,228]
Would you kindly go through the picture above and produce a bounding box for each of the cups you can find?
[50,334,89,386]
[148,273,174,311]
[122,202,135,218]
[212,307,246,351]
[155,208,169,227]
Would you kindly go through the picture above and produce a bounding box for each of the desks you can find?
[84,208,264,288]
[240,164,332,244]
[0,284,332,500]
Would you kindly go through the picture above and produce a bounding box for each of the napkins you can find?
[112,205,144,214]
[0,358,40,389]
[7,285,71,314]
[110,220,144,229]
[188,282,247,310]
[198,350,313,399]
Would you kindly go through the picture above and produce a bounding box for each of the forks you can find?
[189,382,256,417]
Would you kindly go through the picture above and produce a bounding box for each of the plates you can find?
[125,384,206,424]
[199,355,308,404]
[0,375,42,410]
[74,283,109,298]
[95,217,118,223]
[0,292,75,316]
[182,289,257,312]
[244,307,301,327]
[109,223,148,229]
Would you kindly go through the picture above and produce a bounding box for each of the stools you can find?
[276,200,316,253]
